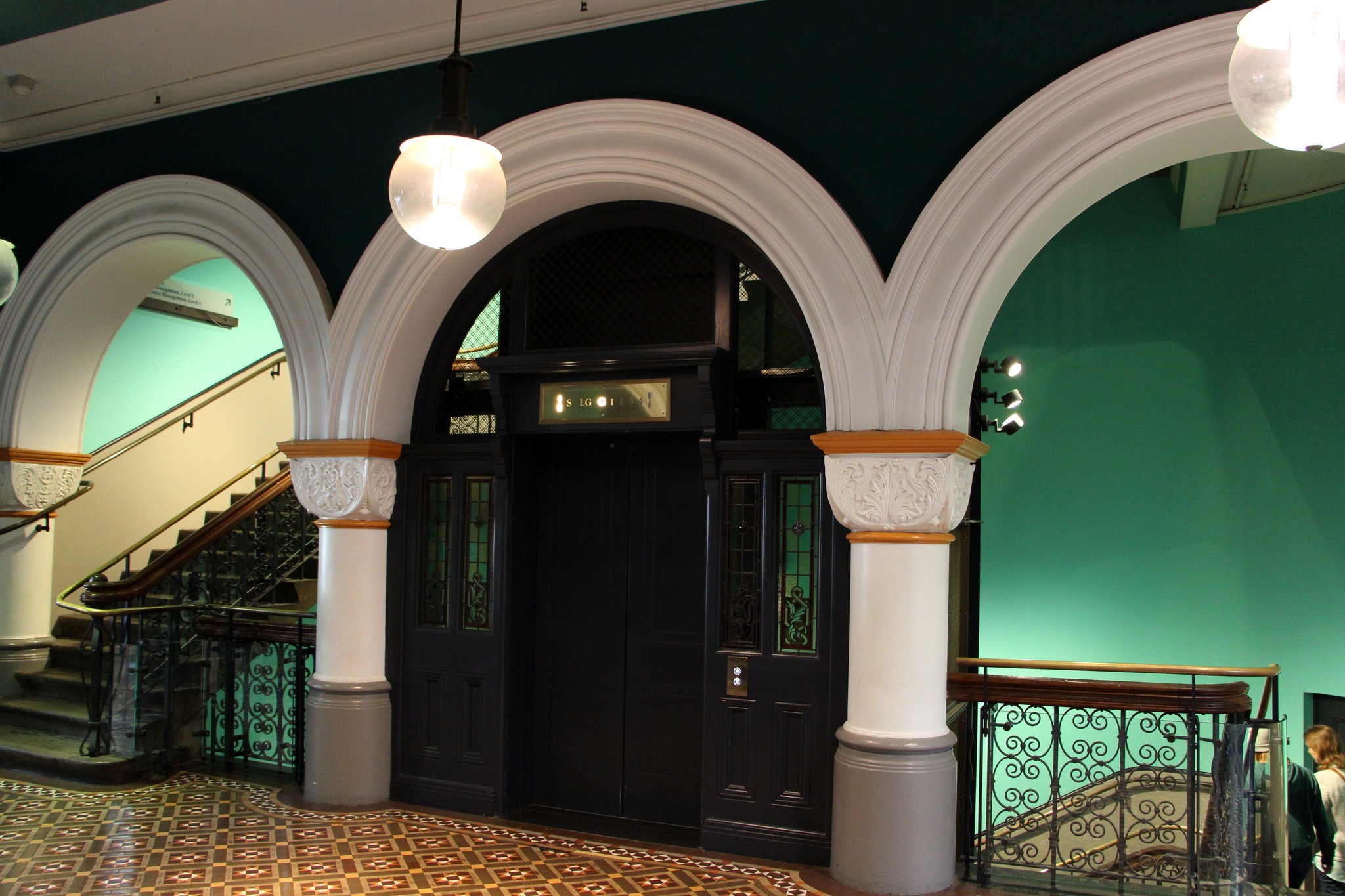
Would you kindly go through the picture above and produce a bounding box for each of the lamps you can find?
[981,413,1024,436]
[388,0,506,253]
[1227,0,1345,154]
[981,386,1023,409]
[981,356,1022,377]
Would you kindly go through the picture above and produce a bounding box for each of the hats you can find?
[1246,723,1270,753]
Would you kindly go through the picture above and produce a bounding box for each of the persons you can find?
[1247,727,1336,889]
[1304,725,1345,896]
